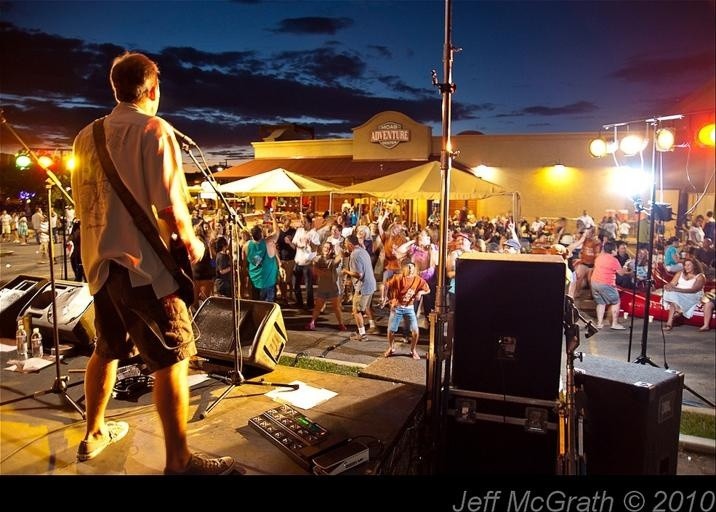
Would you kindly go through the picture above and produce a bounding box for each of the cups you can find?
[681,251,686,258]
[624,312,629,319]
[619,310,624,317]
[649,315,654,322]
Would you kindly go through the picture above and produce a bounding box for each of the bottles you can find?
[15,324,28,356]
[30,326,44,358]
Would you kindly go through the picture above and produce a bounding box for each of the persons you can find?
[0,203,86,283]
[193,198,715,362]
[71,53,236,477]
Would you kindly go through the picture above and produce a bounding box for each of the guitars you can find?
[169,233,194,307]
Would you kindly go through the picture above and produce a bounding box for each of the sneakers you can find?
[76,421,129,461]
[305,323,316,329]
[163,452,236,475]
[337,324,347,330]
[595,324,604,329]
[610,323,625,330]
[349,327,380,342]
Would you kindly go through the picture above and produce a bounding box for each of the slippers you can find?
[672,311,683,320]
[663,324,672,331]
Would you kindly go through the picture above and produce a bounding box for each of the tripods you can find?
[628,126,716,407]
[0,182,87,420]
[197,222,301,420]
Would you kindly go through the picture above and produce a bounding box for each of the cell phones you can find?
[270,208,274,213]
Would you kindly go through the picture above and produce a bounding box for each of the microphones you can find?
[171,126,196,147]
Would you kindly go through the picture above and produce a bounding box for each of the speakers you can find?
[15,279,97,349]
[189,295,289,372]
[442,251,561,400]
[572,352,686,476]
